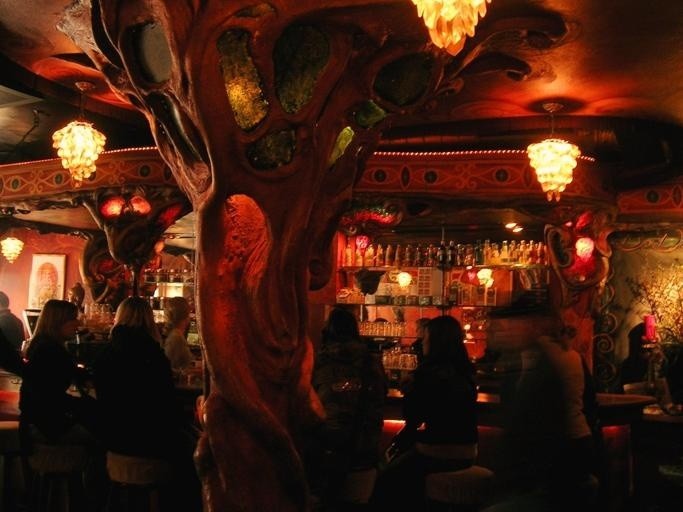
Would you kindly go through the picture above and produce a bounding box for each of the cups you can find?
[356,322,409,337]
[82,303,114,326]
[381,347,416,370]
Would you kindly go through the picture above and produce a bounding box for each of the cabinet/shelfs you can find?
[335,261,548,373]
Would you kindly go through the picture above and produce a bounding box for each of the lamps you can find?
[524,101,581,203]
[1,236,25,266]
[407,0,492,59]
[49,80,108,184]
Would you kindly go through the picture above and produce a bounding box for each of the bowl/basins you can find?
[374,295,440,305]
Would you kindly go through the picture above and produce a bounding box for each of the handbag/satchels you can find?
[395,434,410,461]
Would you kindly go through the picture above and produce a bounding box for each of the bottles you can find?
[342,239,550,266]
[447,281,463,305]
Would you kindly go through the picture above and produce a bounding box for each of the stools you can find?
[312,434,500,511]
[18,434,195,511]
